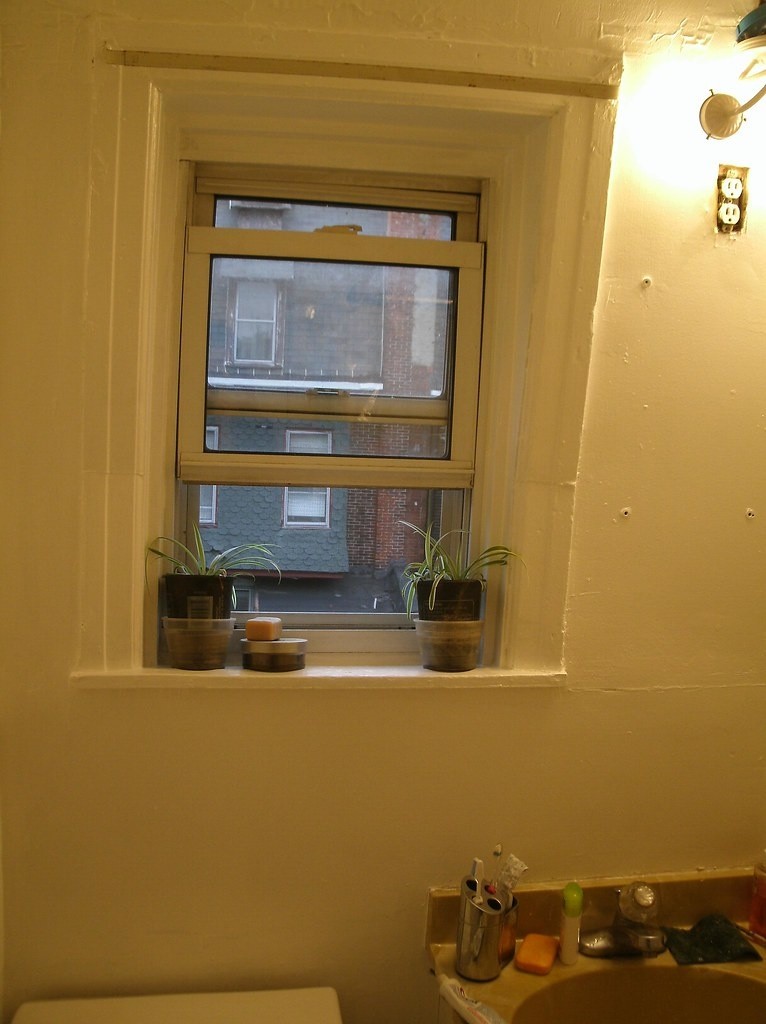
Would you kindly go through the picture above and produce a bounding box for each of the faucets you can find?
[580,881,670,958]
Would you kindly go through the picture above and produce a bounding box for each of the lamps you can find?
[698,0,766,139]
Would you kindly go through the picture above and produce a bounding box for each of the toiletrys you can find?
[559,882,584,966]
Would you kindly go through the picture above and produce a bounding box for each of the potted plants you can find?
[145,520,284,670]
[398,519,528,673]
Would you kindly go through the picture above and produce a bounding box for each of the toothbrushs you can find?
[472,841,504,970]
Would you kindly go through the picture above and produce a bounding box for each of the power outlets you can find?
[717,164,750,235]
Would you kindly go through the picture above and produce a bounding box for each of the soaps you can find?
[246,616,283,640]
[515,932,558,976]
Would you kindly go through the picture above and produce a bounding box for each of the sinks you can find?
[427,919,764,1024]
